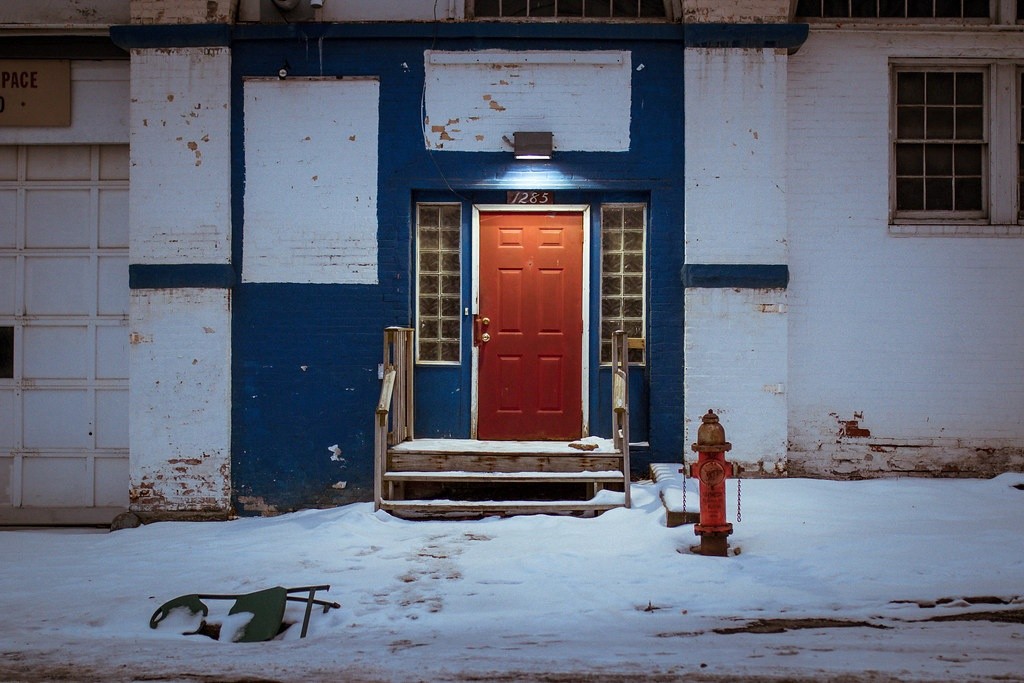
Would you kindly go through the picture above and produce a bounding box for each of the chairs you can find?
[151,583,342,642]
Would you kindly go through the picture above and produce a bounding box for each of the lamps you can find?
[513,131,554,160]
